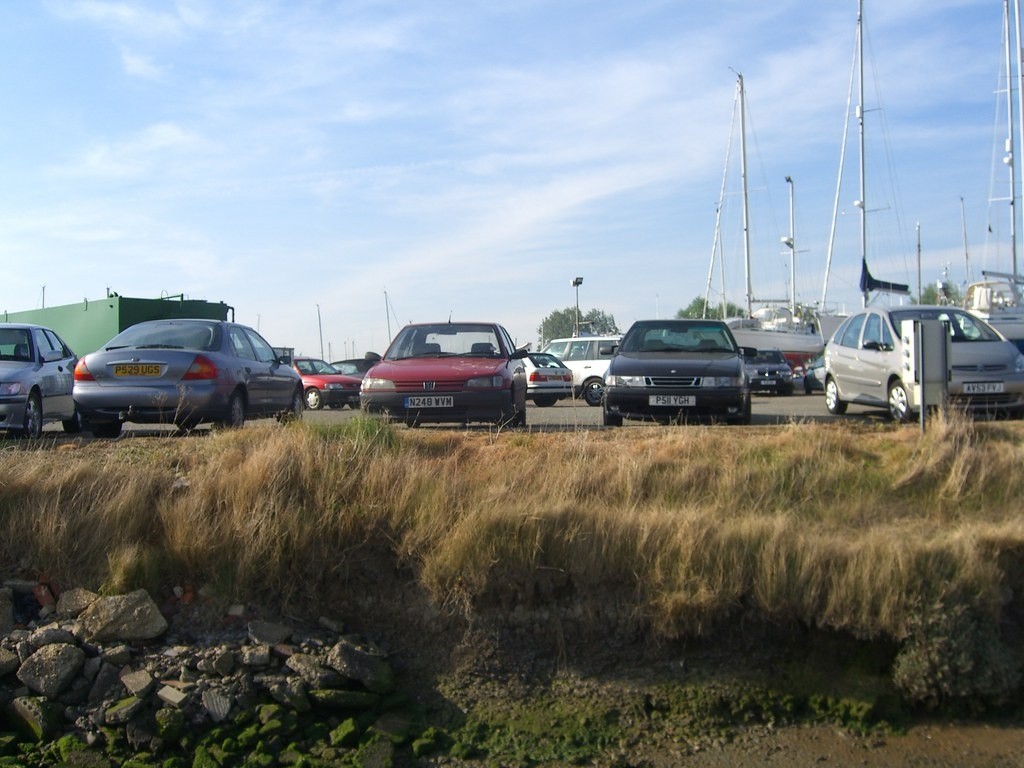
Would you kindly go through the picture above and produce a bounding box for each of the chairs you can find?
[412,343,440,356]
[301,368,310,374]
[14,344,40,360]
[471,343,493,354]
[699,339,718,349]
[644,339,664,348]
[569,348,582,360]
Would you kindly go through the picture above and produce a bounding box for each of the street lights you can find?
[570,277,584,338]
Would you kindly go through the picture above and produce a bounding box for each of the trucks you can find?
[237,347,293,366]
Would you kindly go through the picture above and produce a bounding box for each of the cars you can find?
[360,321,528,430]
[522,352,573,408]
[72,319,305,439]
[803,353,826,395]
[742,348,794,397]
[600,319,758,428]
[825,306,1024,424]
[0,323,82,441]
[290,357,375,411]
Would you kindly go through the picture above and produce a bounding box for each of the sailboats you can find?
[703,0,1023,358]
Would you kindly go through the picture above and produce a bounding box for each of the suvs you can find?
[535,334,624,407]
